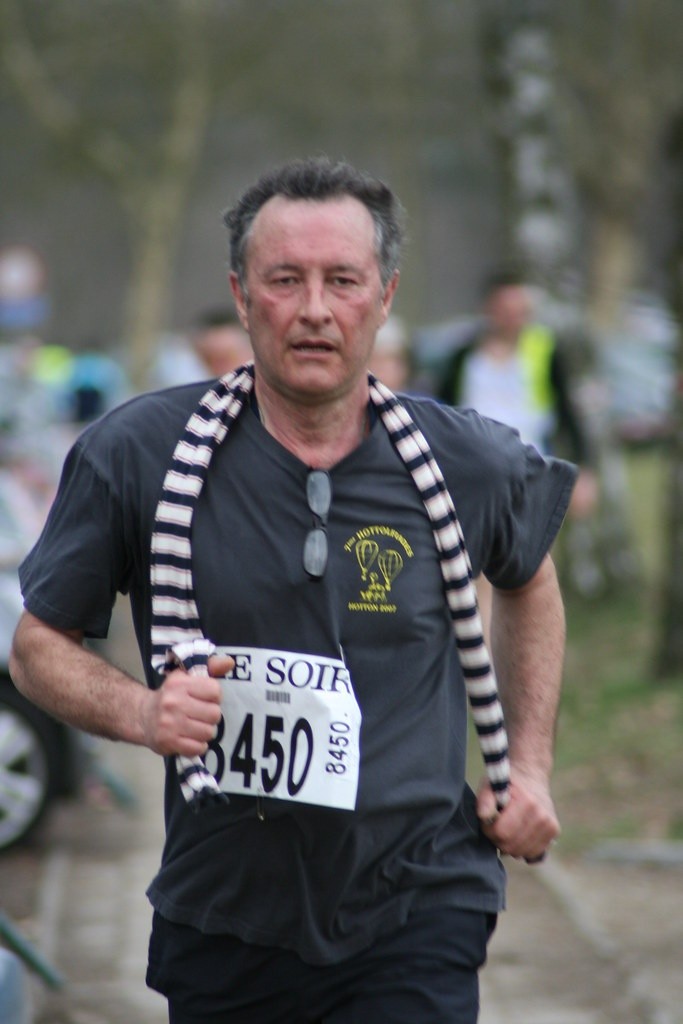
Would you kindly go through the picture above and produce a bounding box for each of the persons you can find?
[430,266,603,525]
[7,155,584,1024]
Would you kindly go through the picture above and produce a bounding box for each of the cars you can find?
[0,295,683,856]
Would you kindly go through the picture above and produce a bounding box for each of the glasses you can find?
[302,466,333,580]
